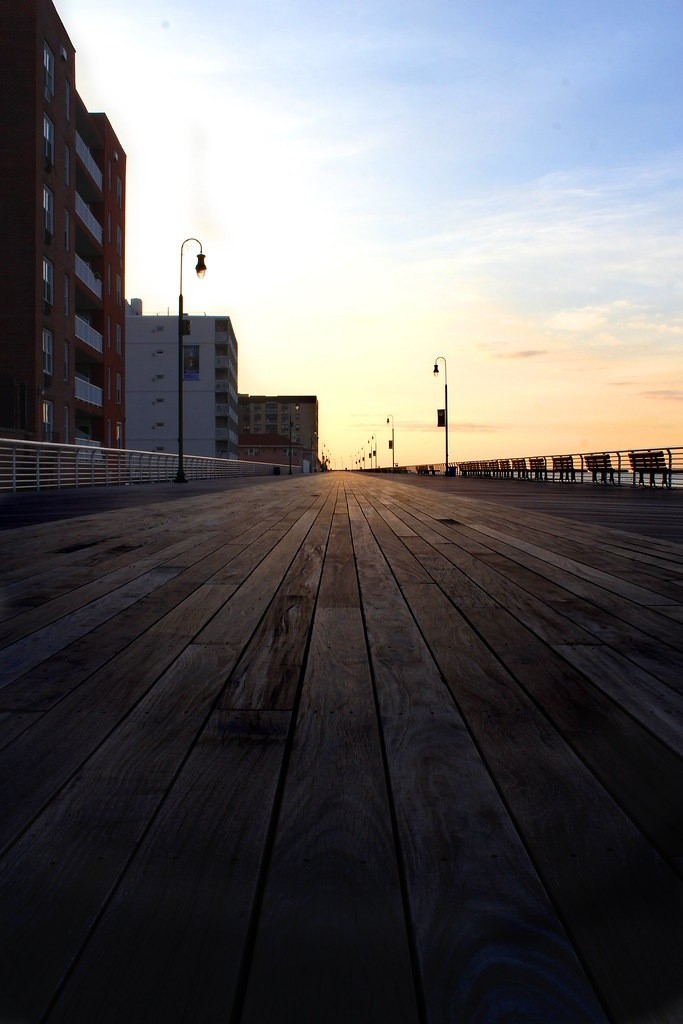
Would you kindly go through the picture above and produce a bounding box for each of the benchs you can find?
[459,457,588,484]
[415,464,440,476]
[584,454,630,487]
[628,451,683,489]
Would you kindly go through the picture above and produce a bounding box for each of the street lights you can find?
[173,238,207,482]
[386,414,394,473]
[433,356,448,474]
[288,398,300,474]
[350,433,377,469]
[309,425,332,473]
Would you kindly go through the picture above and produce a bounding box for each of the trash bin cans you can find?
[274,467,280,475]
[448,467,457,477]
